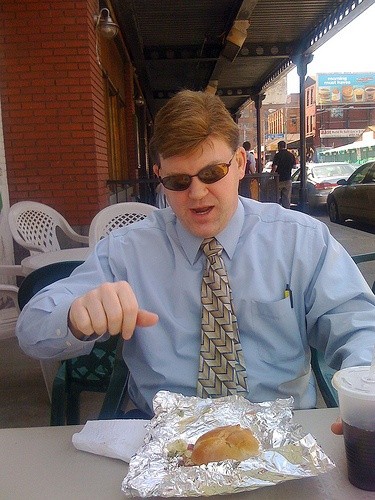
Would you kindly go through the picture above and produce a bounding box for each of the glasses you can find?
[158,149,238,191]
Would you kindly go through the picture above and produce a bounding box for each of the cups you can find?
[330,365,375,491]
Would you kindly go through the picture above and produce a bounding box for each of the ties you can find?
[196,237,250,401]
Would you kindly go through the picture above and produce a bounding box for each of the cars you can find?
[261,161,312,177]
[327,159,375,229]
[293,162,365,214]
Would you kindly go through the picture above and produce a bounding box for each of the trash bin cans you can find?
[239,171,281,204]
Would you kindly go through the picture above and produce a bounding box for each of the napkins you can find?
[72,419,149,463]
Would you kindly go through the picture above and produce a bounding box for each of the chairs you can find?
[88,203,160,254]
[10,201,89,256]
[0,266,56,401]
[311,252,375,407]
[18,260,117,426]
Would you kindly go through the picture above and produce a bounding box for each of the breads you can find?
[191,425,260,465]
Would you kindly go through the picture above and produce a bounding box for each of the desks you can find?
[0,408,375,500]
[21,248,92,277]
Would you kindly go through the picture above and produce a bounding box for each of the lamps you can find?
[221,28,247,63]
[133,90,144,106]
[94,8,120,38]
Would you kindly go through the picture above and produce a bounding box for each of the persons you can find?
[13,87,375,436]
[241,141,263,175]
[271,140,297,209]
